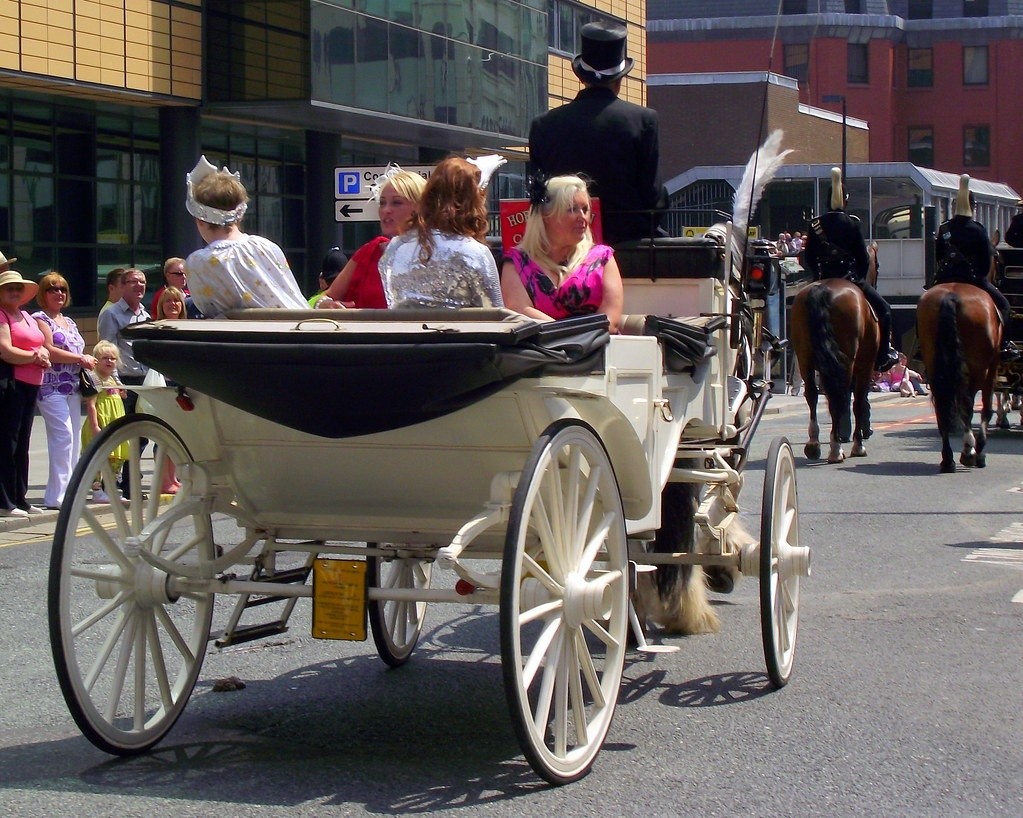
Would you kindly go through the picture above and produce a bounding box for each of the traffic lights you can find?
[745,255,769,296]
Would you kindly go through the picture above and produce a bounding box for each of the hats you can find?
[954,173,975,219]
[570,21,635,85]
[0,251,17,268]
[826,166,848,212]
[0,271,39,307]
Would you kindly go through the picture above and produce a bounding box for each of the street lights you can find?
[822,95,847,182]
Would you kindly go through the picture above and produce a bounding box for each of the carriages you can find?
[994,242,1023,429]
[47,222,813,783]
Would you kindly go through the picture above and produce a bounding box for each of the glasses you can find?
[124,280,145,285]
[169,271,185,276]
[44,287,67,293]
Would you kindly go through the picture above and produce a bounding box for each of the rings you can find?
[42,358,46,363]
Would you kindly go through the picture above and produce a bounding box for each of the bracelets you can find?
[314,296,333,310]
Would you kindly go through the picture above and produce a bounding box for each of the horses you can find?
[791,248,880,463]
[916,228,1003,472]
[520,224,758,643]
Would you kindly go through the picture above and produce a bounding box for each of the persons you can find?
[772,229,810,259]
[526,19,659,277]
[922,190,1011,367]
[498,174,624,336]
[311,166,428,323]
[800,178,899,372]
[0,250,17,279]
[183,154,312,320]
[95,256,190,500]
[79,339,128,503]
[33,271,98,507]
[790,347,929,398]
[378,156,502,325]
[0,270,50,516]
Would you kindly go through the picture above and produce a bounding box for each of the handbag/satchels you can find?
[79,367,102,398]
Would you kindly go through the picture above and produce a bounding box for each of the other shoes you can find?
[117,492,128,502]
[0,507,28,517]
[917,391,929,395]
[26,505,43,514]
[92,489,110,503]
[874,350,898,373]
[1002,343,1020,362]
[172,479,183,488]
[160,482,180,494]
[48,500,64,511]
